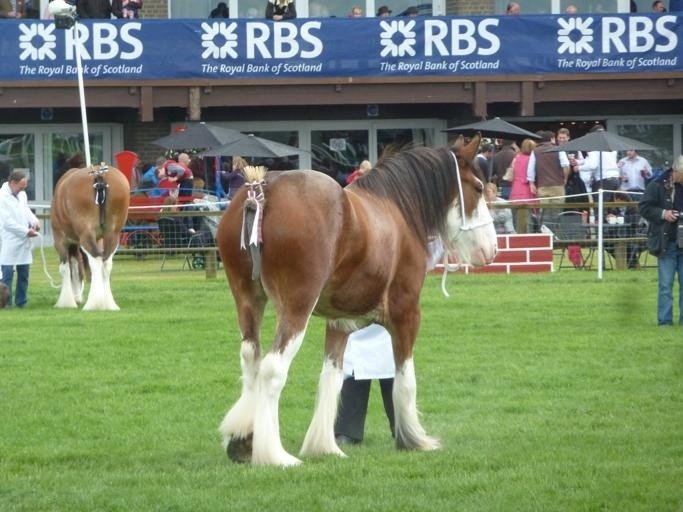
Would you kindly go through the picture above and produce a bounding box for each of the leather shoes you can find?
[335,435,361,445]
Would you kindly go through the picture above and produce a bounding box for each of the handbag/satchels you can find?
[646,220,670,258]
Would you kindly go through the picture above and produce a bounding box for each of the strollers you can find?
[188,199,222,268]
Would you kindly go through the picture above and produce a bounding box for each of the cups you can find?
[606,216,624,225]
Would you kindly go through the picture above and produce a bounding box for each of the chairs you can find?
[554,211,588,271]
[589,213,614,270]
[157,217,204,271]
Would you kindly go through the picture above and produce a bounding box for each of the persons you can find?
[469,124,671,270]
[132,151,248,270]
[345,159,373,184]
[637,155,683,327]
[68,152,87,170]
[1,0,144,25]
[207,0,682,25]
[329,318,399,449]
[0,169,42,309]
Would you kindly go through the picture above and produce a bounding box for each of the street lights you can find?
[48,0,91,167]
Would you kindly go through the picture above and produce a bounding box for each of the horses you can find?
[215,130,500,468]
[48,153,132,311]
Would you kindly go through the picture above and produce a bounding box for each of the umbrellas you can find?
[540,130,657,280]
[144,120,249,193]
[438,116,545,183]
[189,133,310,170]
